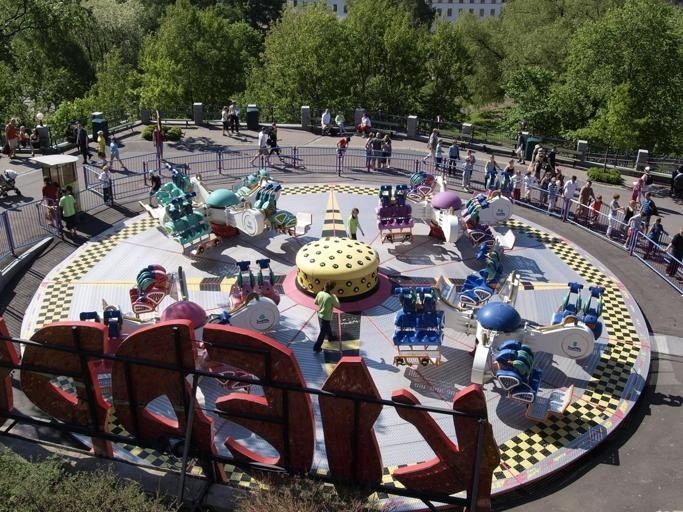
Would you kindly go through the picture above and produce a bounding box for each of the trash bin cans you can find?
[525,138,540,161]
[246,108,259,130]
[92,118,109,141]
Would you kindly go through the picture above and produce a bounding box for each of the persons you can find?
[606,166,682,277]
[228,101,239,134]
[320,108,333,136]
[220,105,233,138]
[4,116,124,236]
[153,124,165,159]
[484,131,603,228]
[364,131,392,169]
[313,278,341,353]
[148,169,161,206]
[354,113,371,138]
[249,123,284,167]
[334,111,346,133]
[336,136,351,155]
[347,208,364,240]
[423,128,476,192]
